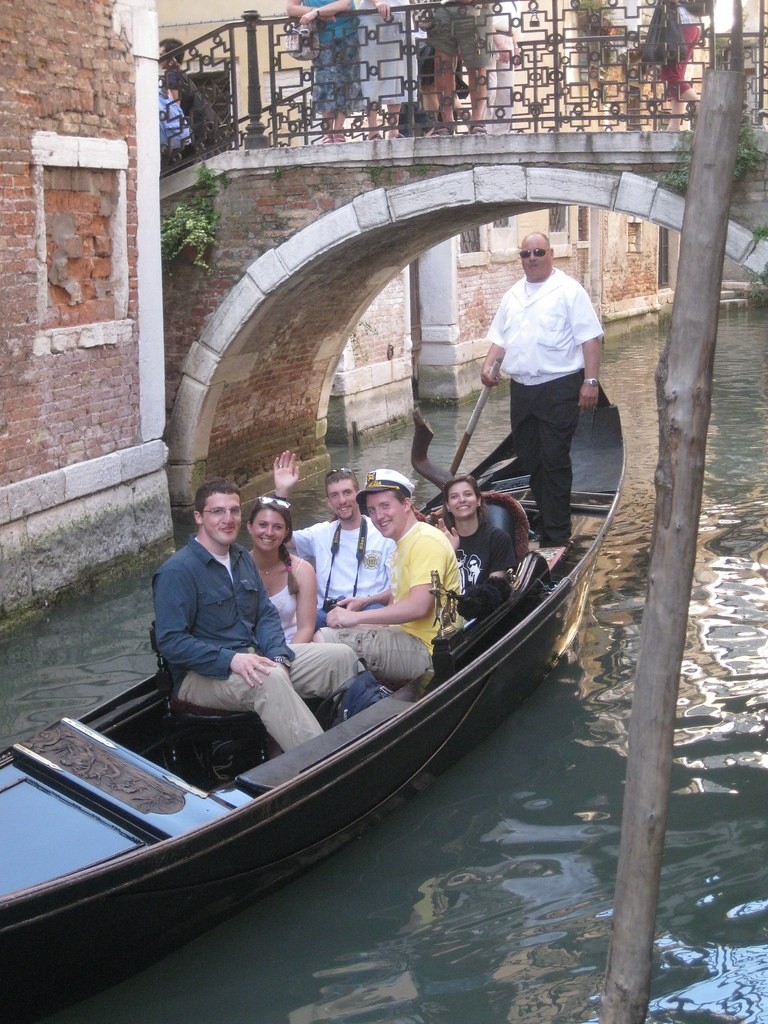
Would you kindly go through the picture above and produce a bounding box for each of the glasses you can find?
[196,508,242,516]
[253,496,291,513]
[520,248,550,258]
[326,467,356,481]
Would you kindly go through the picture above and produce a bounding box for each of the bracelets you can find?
[317,9,320,15]
[514,46,518,48]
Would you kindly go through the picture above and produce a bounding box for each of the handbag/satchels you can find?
[642,0,689,64]
[286,18,318,61]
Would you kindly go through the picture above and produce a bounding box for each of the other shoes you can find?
[333,133,345,143]
[430,125,453,135]
[529,532,570,548]
[470,123,487,136]
[322,135,333,144]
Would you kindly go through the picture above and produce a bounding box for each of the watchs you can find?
[273,657,291,669]
[584,379,598,386]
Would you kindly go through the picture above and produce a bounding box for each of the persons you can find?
[248,497,316,643]
[160,39,223,161]
[480,233,605,547]
[286,0,360,144]
[312,469,462,685]
[659,0,701,131]
[154,481,364,754]
[274,450,395,630]
[434,474,514,591]
[360,0,417,141]
[399,0,521,137]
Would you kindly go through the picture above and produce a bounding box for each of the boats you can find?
[0,378,631,1016]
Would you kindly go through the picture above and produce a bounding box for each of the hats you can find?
[355,469,415,507]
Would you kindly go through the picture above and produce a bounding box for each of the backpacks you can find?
[314,657,393,731]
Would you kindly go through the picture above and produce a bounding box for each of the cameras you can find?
[322,595,347,612]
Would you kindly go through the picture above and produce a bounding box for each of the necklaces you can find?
[265,572,268,574]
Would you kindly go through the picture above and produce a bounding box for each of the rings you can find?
[279,466,283,468]
